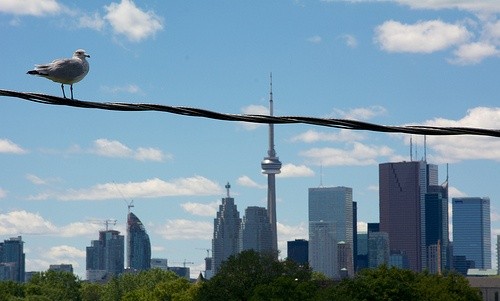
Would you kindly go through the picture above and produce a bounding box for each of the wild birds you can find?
[26,48,91,101]
